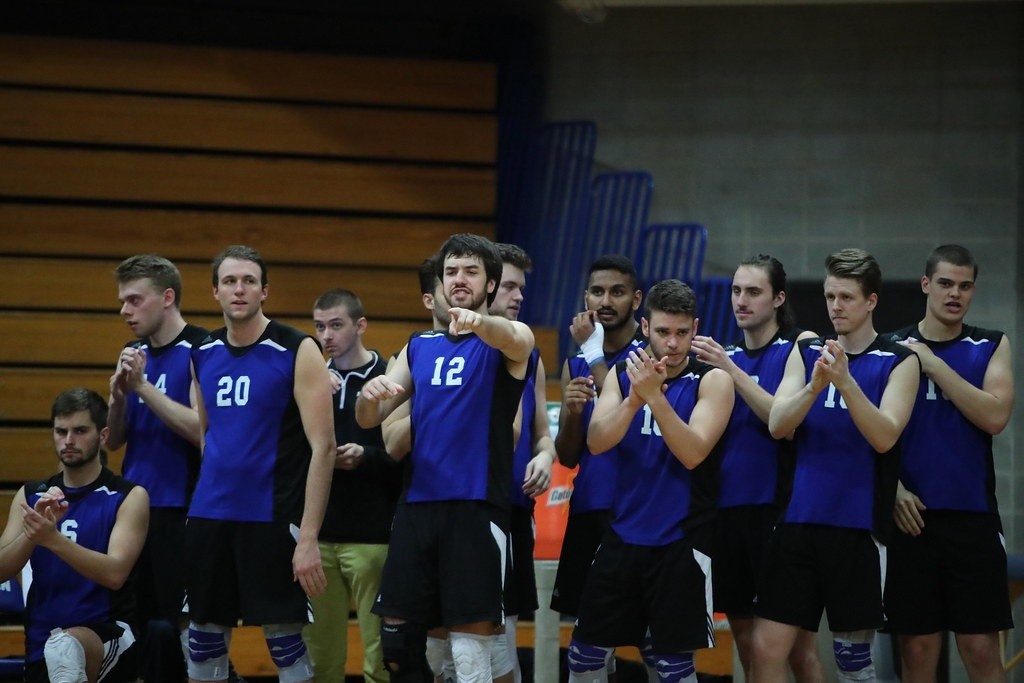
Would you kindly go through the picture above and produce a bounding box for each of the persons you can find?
[548,244,1018,683]
[0,234,557,683]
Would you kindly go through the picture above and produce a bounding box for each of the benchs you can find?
[0,27,729,678]
